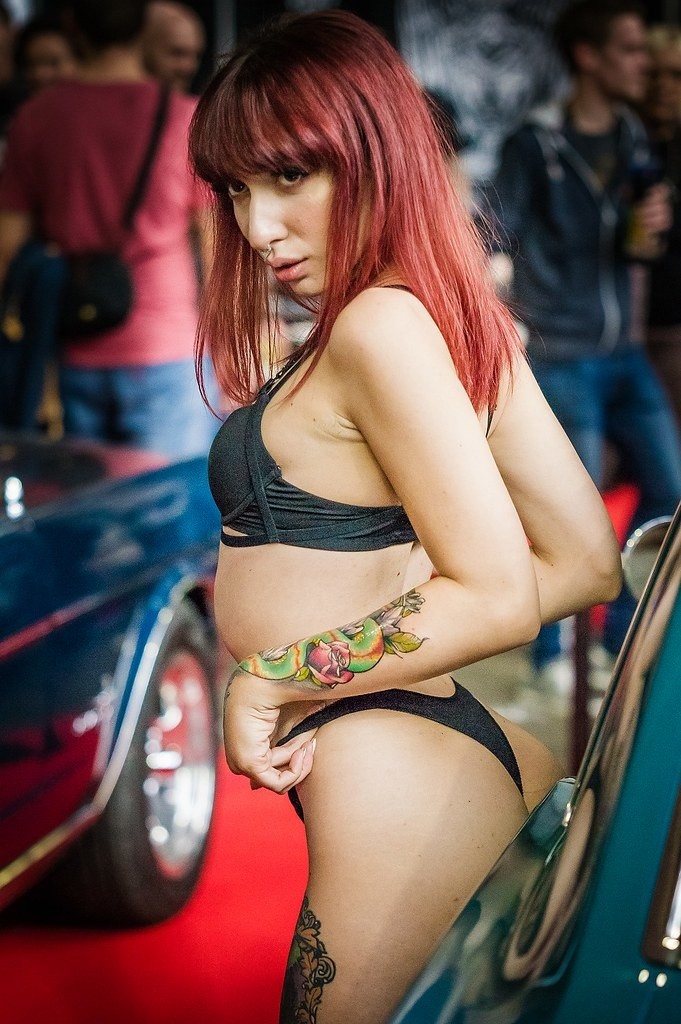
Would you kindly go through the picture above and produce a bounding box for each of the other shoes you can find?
[542,645,613,723]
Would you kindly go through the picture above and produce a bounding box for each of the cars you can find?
[384,499,680,1024]
[0,445,222,931]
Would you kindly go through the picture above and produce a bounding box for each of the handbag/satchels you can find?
[24,246,131,352]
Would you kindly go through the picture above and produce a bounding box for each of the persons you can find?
[187,14,627,1024]
[0,0,681,725]
